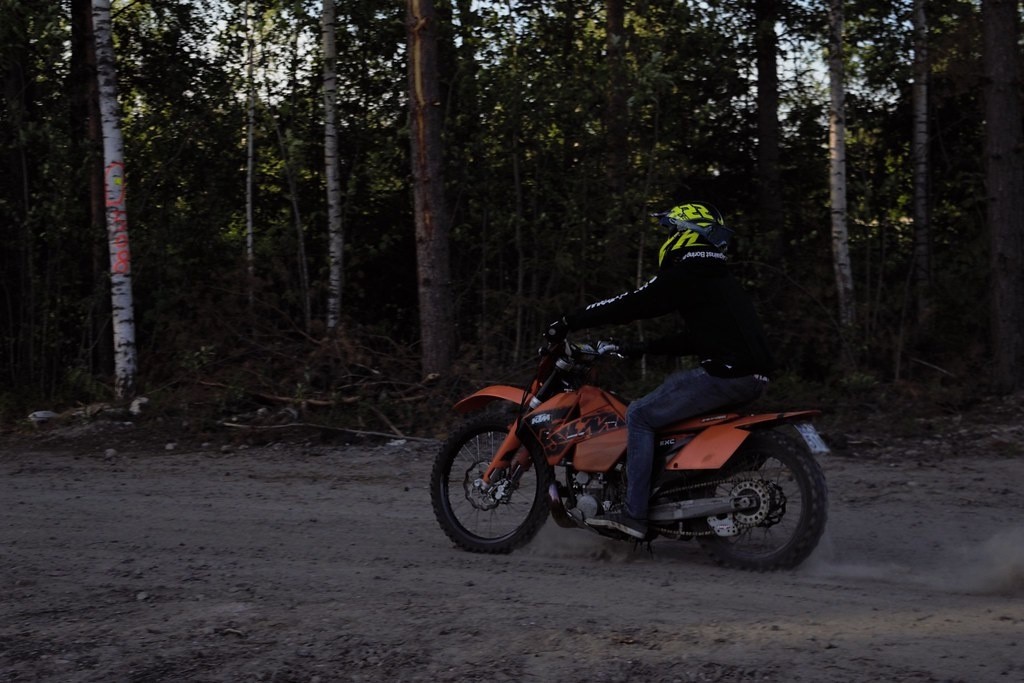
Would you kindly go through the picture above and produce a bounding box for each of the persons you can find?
[546,202,773,540]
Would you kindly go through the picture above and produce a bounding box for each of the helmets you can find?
[657,201,728,266]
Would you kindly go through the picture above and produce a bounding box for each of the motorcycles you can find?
[428,316,832,572]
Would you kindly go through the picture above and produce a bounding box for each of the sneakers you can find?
[585,508,648,540]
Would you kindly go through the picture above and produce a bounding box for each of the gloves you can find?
[544,319,568,342]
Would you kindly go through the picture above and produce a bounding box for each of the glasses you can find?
[680,222,734,248]
